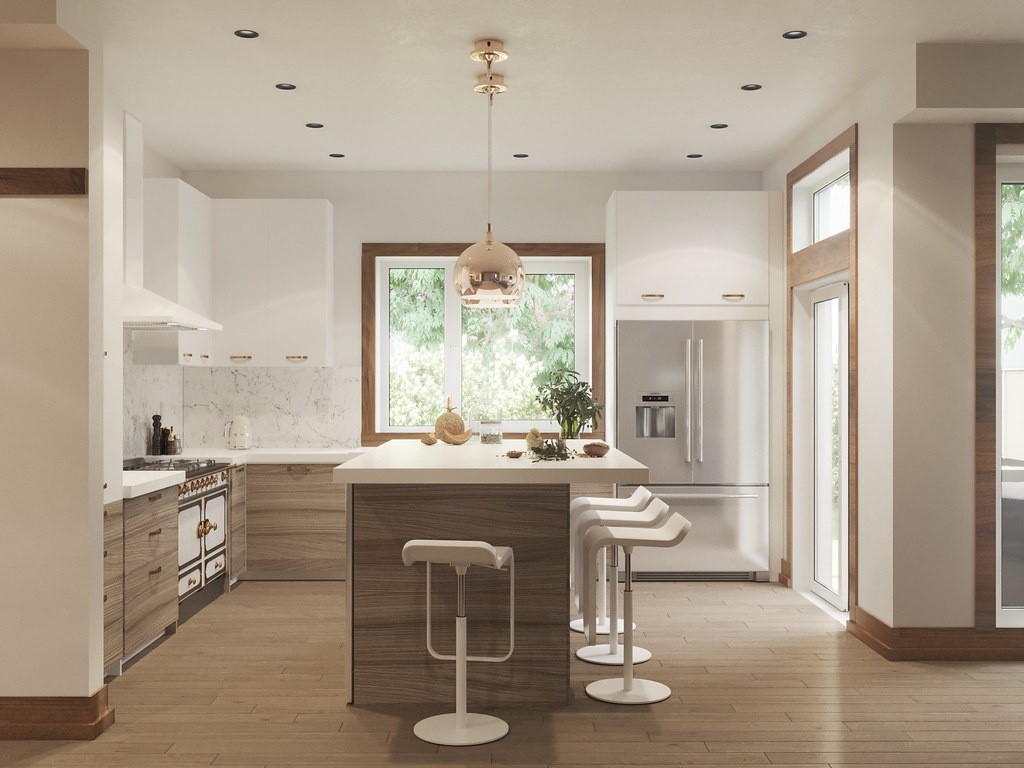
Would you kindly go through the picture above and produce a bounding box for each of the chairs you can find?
[570,485,692,705]
[402,538,514,748]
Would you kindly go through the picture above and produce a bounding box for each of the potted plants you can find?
[538,356,606,440]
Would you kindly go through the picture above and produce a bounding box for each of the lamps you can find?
[454,40,526,310]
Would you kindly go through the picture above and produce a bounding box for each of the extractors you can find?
[123,285,223,331]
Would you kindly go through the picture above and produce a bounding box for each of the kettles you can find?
[224,416,253,450]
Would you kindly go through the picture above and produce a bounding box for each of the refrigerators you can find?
[617,319,770,583]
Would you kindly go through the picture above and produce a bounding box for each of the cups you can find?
[480,421,502,444]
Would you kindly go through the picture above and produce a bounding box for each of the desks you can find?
[333,438,650,704]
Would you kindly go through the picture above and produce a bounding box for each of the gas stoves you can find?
[123,457,234,500]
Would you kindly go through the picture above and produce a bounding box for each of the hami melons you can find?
[441,429,473,444]
[421,432,437,445]
[435,412,464,439]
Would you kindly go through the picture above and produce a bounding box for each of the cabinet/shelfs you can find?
[227,464,245,590]
[102,483,180,675]
[606,190,771,582]
[246,465,348,583]
[130,176,334,369]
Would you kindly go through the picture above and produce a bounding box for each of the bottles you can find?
[173,434,182,455]
[467,392,501,446]
